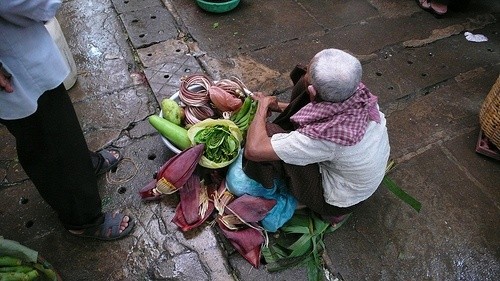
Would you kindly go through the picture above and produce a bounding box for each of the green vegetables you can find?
[261,212,353,281]
[194,96,258,163]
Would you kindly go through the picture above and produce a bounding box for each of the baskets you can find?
[479,76,500,151]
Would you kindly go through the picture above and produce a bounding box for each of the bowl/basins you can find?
[158,80,255,154]
[196,0,240,13]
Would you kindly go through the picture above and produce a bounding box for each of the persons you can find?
[242,47,391,223]
[418,0,448,19]
[0,0,134,240]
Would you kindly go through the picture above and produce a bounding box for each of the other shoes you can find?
[418,0,447,18]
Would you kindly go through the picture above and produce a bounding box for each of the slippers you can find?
[95,147,123,178]
[67,211,135,241]
